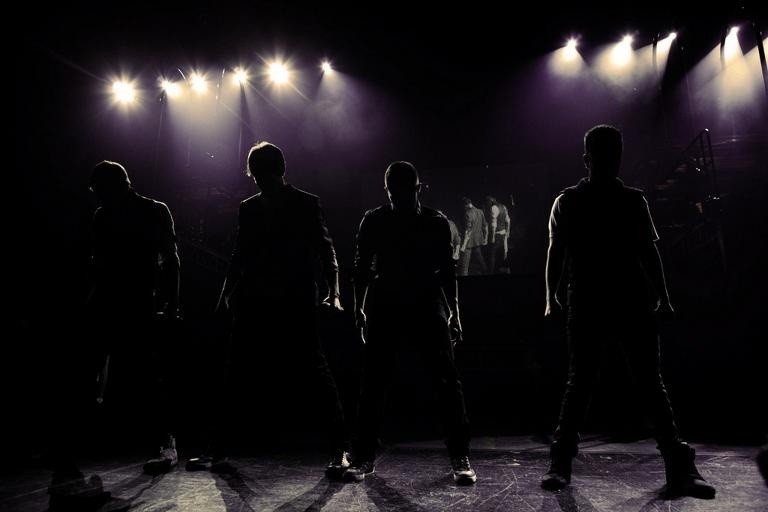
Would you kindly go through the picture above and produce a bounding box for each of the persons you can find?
[481,198,510,274]
[459,197,487,276]
[186,141,346,476]
[58,161,181,469]
[342,159,477,485]
[438,208,461,276]
[541,125,717,500]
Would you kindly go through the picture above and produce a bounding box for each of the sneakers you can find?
[327,452,375,481]
[452,455,476,484]
[142,438,178,472]
[662,441,716,498]
[541,440,574,489]
